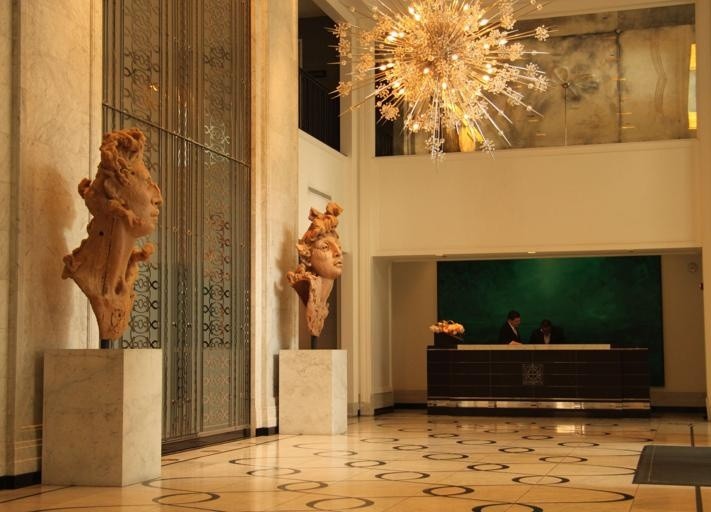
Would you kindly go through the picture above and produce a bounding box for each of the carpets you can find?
[629,446,710,490]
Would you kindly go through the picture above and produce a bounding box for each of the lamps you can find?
[325,0,558,156]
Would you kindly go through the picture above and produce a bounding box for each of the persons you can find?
[500,310,523,344]
[61,127,163,341]
[530,319,561,344]
[285,202,344,337]
